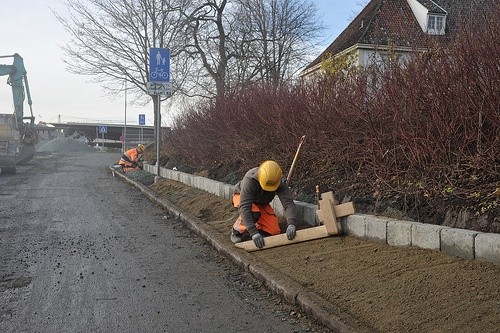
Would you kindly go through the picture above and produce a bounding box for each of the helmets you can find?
[257,160,282,191]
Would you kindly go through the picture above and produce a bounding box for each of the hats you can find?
[138,144,146,152]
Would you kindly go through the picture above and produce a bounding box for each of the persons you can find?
[231,161,299,249]
[119,144,146,173]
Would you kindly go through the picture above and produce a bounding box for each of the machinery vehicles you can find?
[0,53,39,173]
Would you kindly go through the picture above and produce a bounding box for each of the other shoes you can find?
[230,228,243,243]
[119,165,125,175]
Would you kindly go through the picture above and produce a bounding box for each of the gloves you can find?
[286,224,295,240]
[252,232,265,249]
